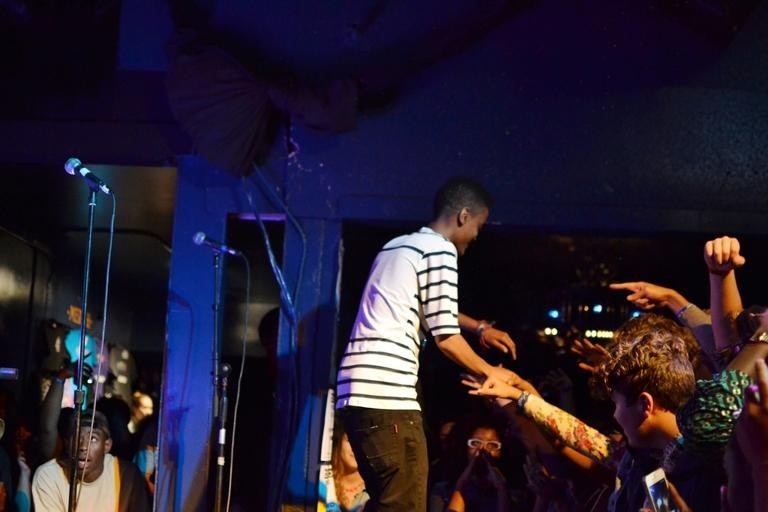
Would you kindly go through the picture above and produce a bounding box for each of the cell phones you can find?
[641,467,680,512]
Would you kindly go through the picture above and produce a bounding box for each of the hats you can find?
[69,408,111,438]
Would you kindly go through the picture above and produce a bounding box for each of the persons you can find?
[216,299,283,511]
[335,175,519,512]
[0,353,157,511]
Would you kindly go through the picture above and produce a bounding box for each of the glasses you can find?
[467,438,502,452]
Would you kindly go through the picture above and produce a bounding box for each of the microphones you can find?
[193,232,242,256]
[64,157,115,197]
